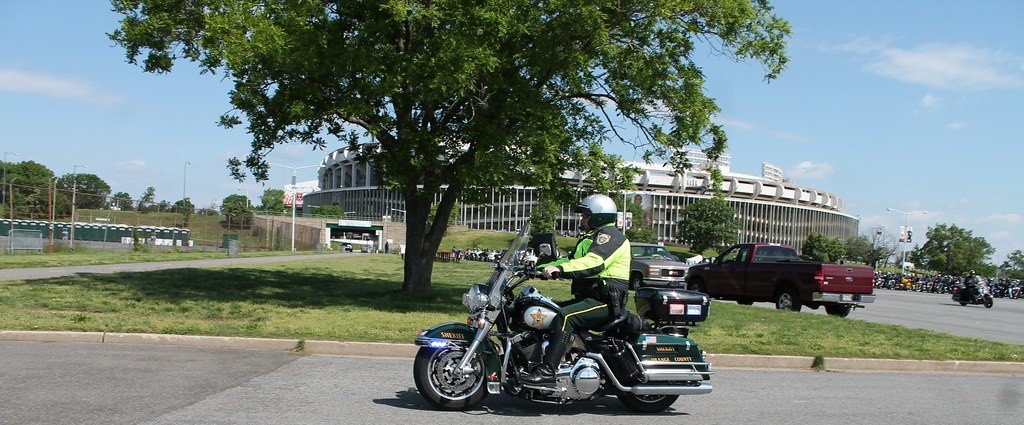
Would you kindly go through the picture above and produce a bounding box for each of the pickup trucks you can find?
[685,241,876,317]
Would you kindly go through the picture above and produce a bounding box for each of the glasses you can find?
[581,214,591,218]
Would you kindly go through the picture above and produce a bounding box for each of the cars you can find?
[628,241,690,290]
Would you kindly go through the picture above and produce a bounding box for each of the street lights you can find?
[391,209,406,228]
[265,162,326,252]
[73,165,87,182]
[886,207,929,274]
[3,152,18,207]
[184,161,191,207]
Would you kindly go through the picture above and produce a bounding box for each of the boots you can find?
[519,332,572,388]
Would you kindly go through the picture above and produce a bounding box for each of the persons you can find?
[385,242,388,254]
[874,270,1024,299]
[963,270,980,290]
[464,246,507,263]
[517,193,631,388]
[452,244,463,261]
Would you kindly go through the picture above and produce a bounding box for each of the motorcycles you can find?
[413,220,715,414]
[949,275,993,309]
[873,273,1024,300]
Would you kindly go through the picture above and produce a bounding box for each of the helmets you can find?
[968,270,975,274]
[574,194,618,229]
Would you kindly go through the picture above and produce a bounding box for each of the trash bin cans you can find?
[228,239,241,256]
[222,233,238,248]
[0,218,191,246]
